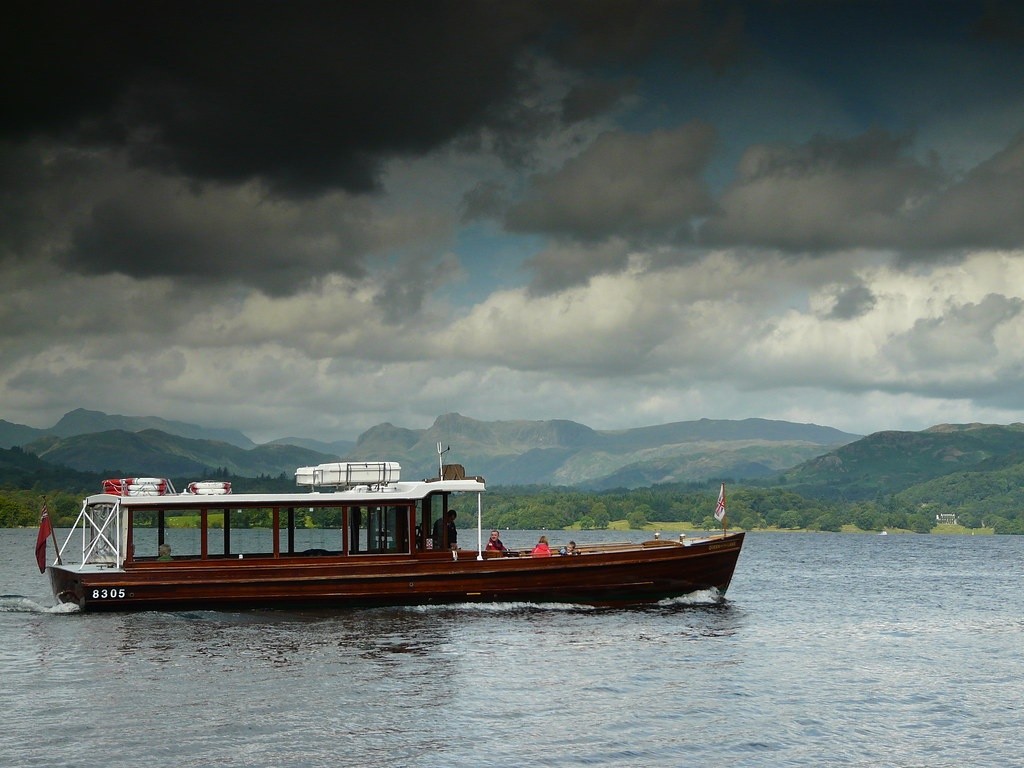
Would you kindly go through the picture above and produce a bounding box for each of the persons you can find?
[485,531,506,550]
[433,510,457,550]
[559,541,581,556]
[532,536,551,557]
[132,544,136,561]
[156,544,173,561]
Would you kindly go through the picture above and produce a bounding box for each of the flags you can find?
[35,502,52,574]
[713,483,726,523]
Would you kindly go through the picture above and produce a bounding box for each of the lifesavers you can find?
[101,477,168,497]
[188,480,233,496]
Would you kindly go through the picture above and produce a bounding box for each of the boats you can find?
[35,442,745,613]
